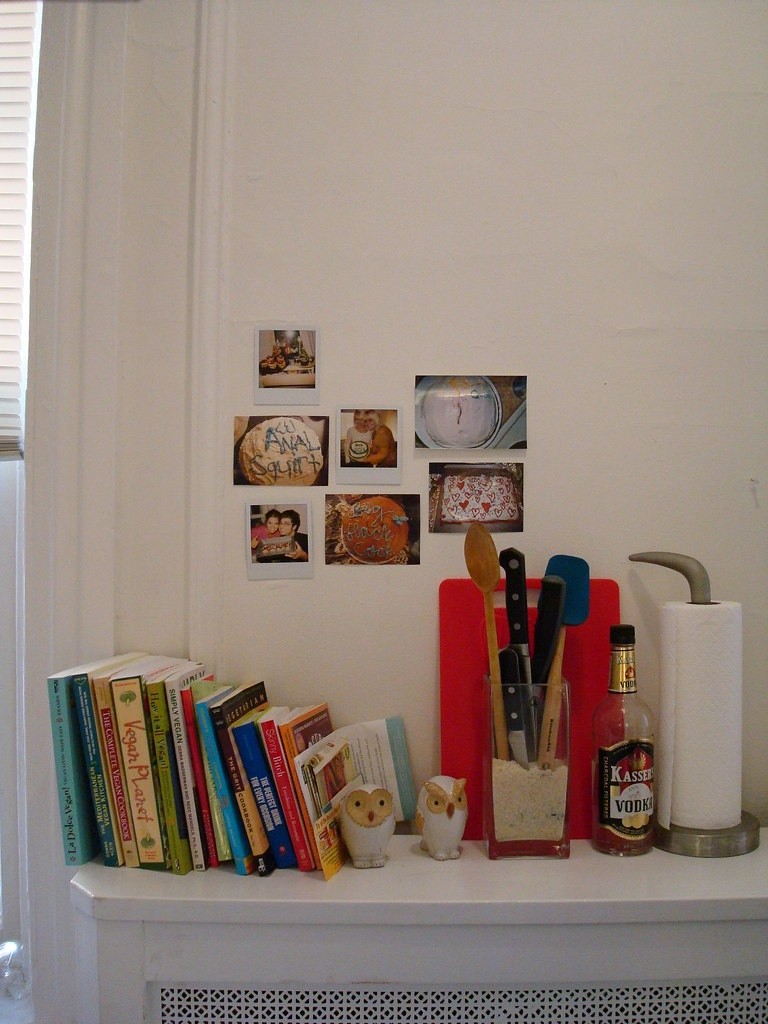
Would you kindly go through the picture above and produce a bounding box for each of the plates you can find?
[415,375,502,450]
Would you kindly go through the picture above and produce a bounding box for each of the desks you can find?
[70,827,768,1024]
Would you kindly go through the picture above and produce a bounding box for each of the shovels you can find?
[538,554,590,771]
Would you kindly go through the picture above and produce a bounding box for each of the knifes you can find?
[500,649,528,770]
[532,575,567,719]
[499,547,537,763]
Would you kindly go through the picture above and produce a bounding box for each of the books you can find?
[48,653,333,876]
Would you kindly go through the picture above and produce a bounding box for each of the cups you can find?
[490,684,570,860]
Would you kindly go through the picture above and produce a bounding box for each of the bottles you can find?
[592,625,654,857]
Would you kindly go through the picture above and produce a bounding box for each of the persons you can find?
[251,509,308,563]
[343,410,395,468]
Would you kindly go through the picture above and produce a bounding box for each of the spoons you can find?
[464,523,508,761]
